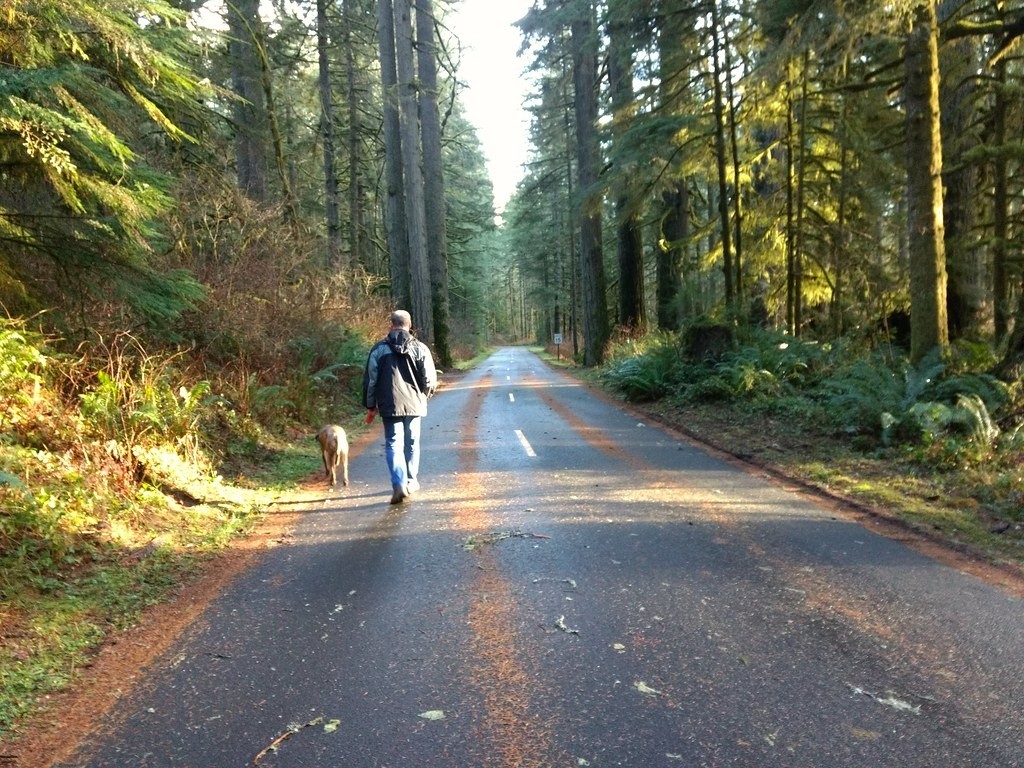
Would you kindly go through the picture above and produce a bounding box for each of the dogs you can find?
[313,424,350,487]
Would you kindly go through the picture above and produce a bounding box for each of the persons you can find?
[362,310,437,505]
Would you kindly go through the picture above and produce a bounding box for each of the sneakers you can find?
[390,482,421,505]
